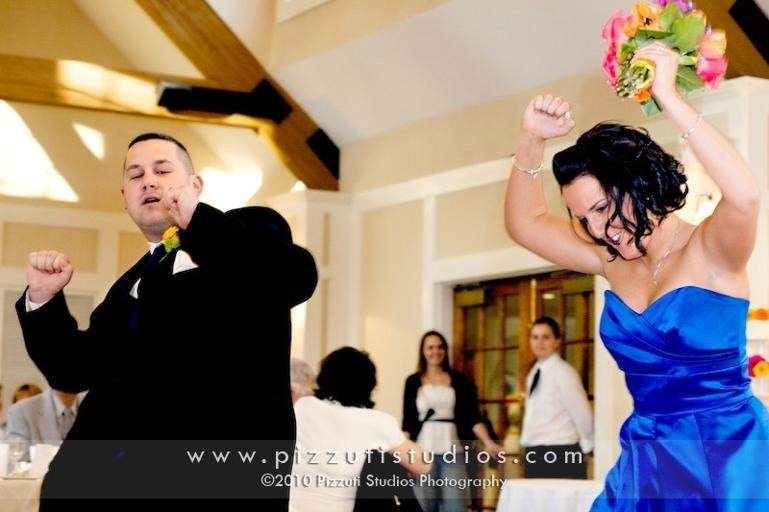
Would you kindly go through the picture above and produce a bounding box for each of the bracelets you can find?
[679,108,702,142]
[508,150,547,177]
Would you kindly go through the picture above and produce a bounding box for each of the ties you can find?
[129,244,163,336]
[530,369,540,396]
[63,409,72,439]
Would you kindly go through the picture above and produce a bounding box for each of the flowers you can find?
[747,355,769,378]
[599,0,729,117]
[161,226,181,253]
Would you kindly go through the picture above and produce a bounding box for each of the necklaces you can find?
[639,218,682,288]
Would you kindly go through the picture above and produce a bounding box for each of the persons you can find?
[0,132,506,512]
[401,331,500,512]
[519,318,595,477]
[5,383,83,477]
[502,39,768,512]
[289,345,435,512]
